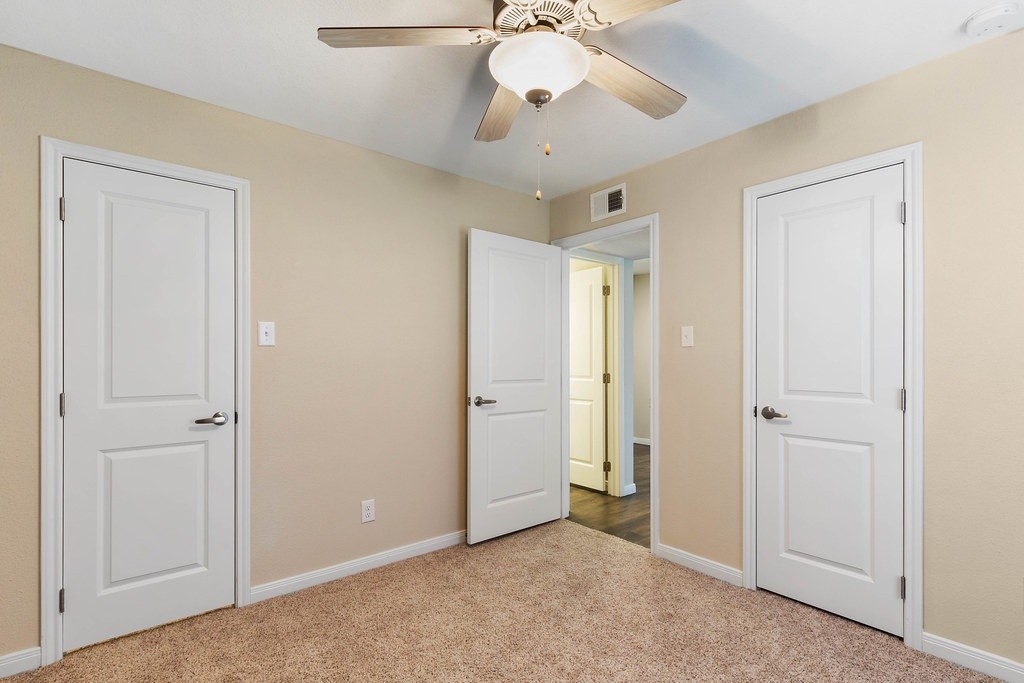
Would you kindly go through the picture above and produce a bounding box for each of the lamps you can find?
[488,31,591,201]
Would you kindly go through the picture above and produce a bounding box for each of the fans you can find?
[317,1,687,143]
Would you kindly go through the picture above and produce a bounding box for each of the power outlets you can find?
[361,499,376,523]
